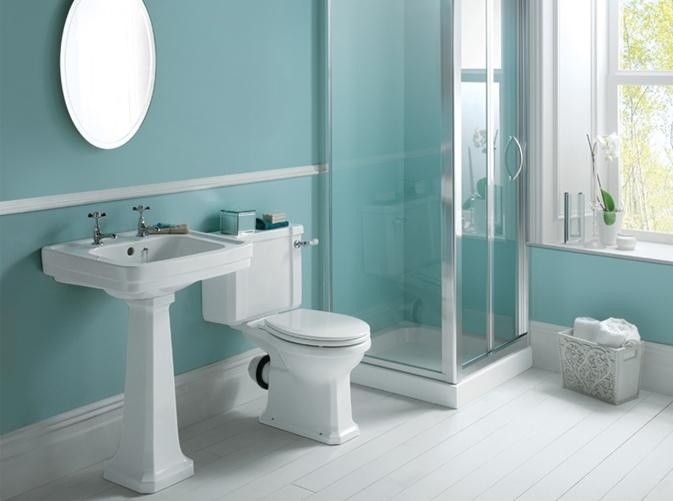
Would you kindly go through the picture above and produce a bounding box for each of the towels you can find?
[574,316,638,347]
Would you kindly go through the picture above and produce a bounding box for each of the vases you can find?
[471,199,486,232]
[597,210,622,246]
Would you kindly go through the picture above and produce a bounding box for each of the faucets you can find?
[132,203,160,236]
[88,211,118,244]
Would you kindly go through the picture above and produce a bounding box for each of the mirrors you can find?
[59,0,156,150]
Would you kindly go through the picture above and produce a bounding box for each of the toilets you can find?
[201,224,372,446]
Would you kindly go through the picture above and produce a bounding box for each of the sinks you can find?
[41,225,255,302]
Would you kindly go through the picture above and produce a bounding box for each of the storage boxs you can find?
[558,328,644,405]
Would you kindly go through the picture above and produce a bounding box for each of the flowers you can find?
[463,129,487,210]
[586,132,622,225]
